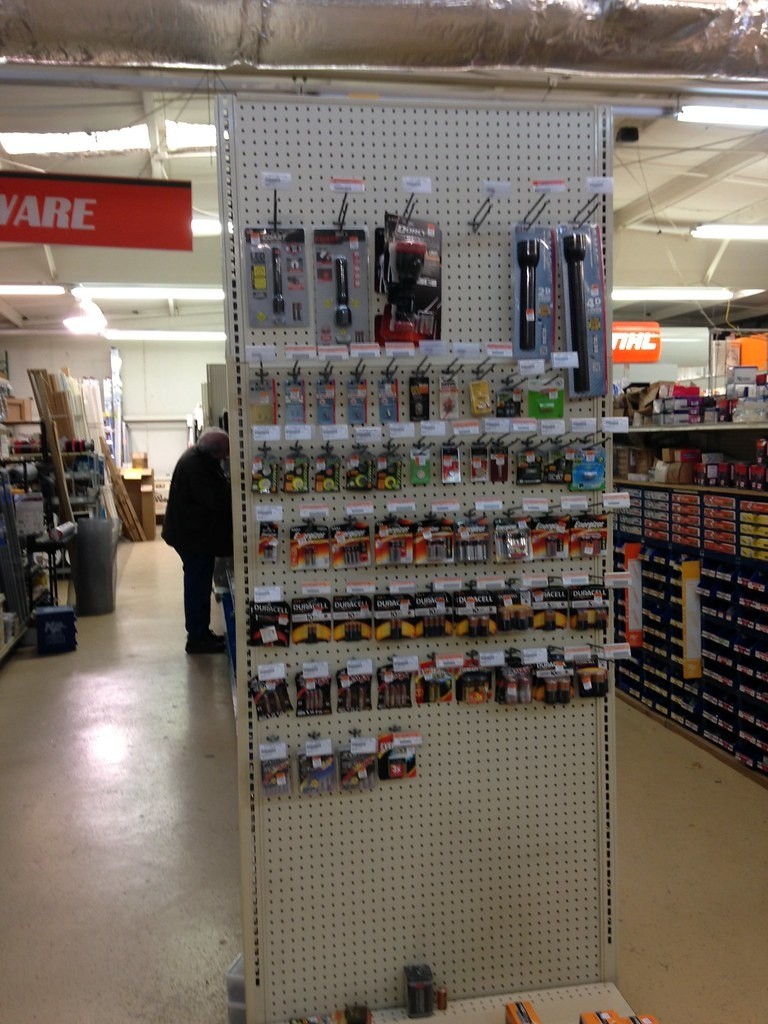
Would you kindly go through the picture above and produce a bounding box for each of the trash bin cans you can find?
[71,520,117,616]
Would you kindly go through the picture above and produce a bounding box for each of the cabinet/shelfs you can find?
[47,451,101,525]
[0,456,46,663]
[612,422,768,791]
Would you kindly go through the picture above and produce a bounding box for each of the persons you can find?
[162,427,234,655]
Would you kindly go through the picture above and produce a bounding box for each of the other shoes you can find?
[185,630,226,654]
